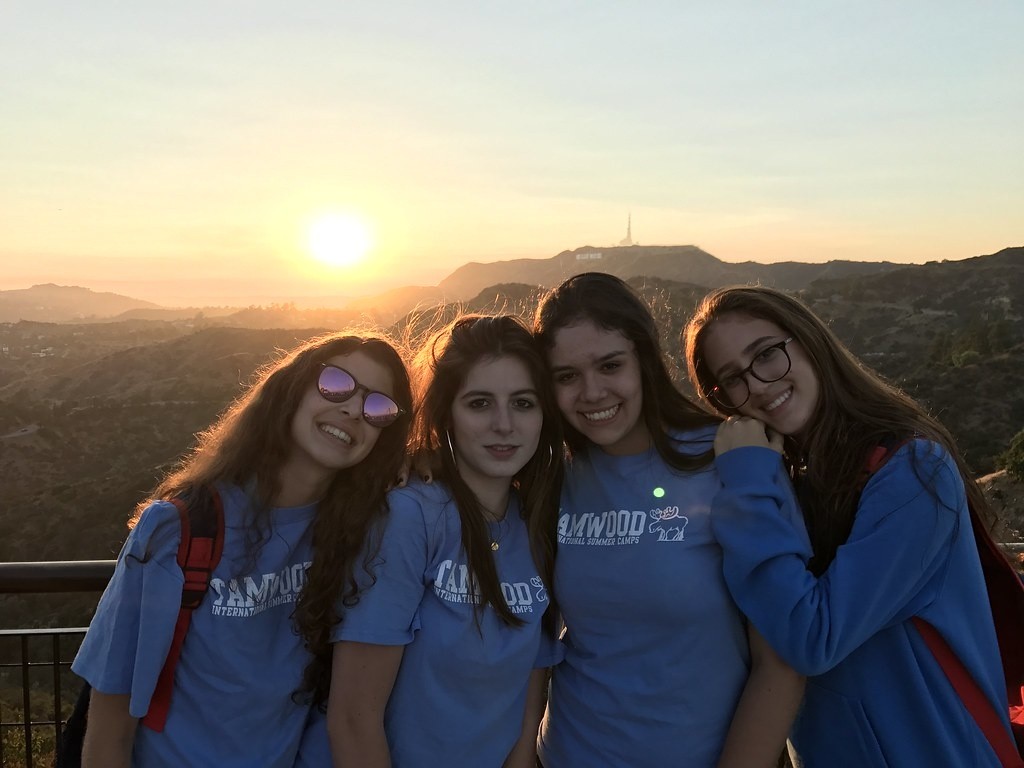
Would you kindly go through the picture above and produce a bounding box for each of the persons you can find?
[327,313,567,768]
[384,272,807,768]
[681,285,1024,768]
[70,332,413,768]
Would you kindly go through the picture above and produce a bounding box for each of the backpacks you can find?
[51,477,224,768]
[853,433,1024,768]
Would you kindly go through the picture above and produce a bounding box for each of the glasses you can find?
[316,362,407,428]
[705,335,795,409]
